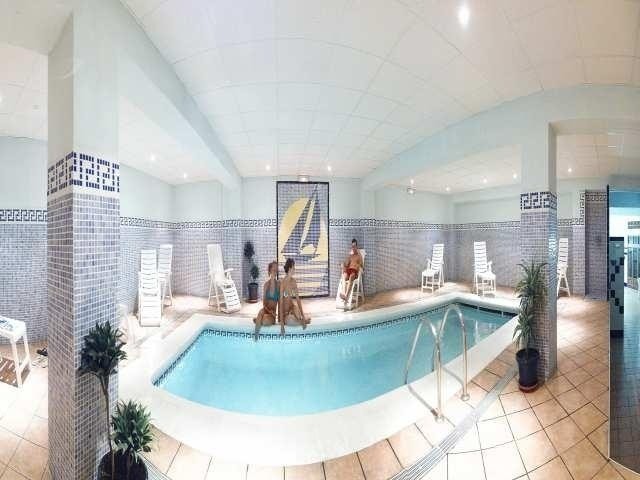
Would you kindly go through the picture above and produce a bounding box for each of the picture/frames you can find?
[275,180,332,301]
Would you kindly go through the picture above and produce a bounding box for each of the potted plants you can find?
[76,322,157,480]
[507,253,552,393]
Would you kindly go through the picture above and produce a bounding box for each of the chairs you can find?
[205,242,241,315]
[156,243,177,316]
[135,246,162,329]
[473,240,498,300]
[557,238,571,300]
[0,314,33,389]
[421,242,446,296]
[334,247,368,311]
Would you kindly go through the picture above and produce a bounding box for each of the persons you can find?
[279,258,310,336]
[340,238,364,301]
[253,262,279,334]
